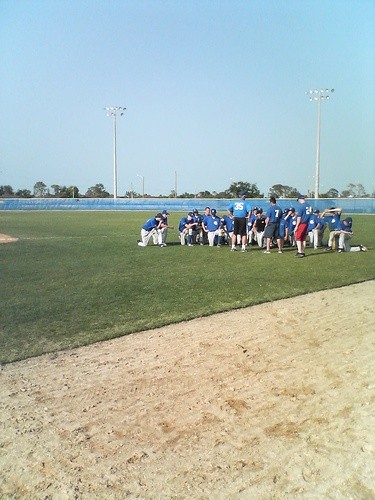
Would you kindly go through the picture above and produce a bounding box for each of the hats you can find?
[345,217,352,225]
[211,209,216,214]
[193,209,198,213]
[228,207,233,212]
[188,212,193,217]
[254,207,257,210]
[162,210,168,214]
[289,207,295,213]
[258,208,262,212]
[157,213,163,217]
[297,195,304,201]
[284,209,289,213]
[240,191,247,195]
[314,210,319,213]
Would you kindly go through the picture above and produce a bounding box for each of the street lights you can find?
[105,106,127,198]
[304,88,335,199]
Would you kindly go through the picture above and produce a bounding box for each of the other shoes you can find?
[164,244,167,247]
[327,247,332,251]
[188,244,323,248]
[137,240,141,243]
[278,250,282,253]
[294,253,305,257]
[337,248,343,253]
[242,249,248,252]
[264,250,271,254]
[231,249,237,251]
[359,244,368,252]
[160,244,163,247]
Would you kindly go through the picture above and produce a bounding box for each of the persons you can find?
[294,196,313,257]
[229,196,252,253]
[265,198,284,255]
[136,207,368,253]
